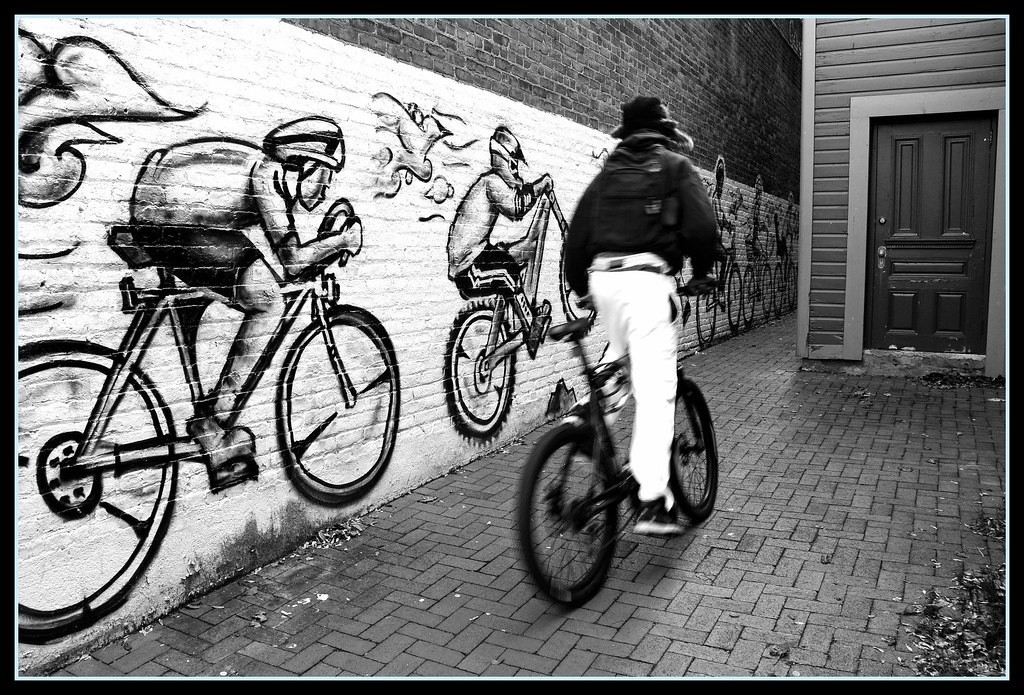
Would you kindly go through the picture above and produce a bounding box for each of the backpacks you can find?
[591,144,676,251]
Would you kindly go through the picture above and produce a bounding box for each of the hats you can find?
[609,96,677,139]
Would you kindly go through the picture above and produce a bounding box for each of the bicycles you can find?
[515,277,720,611]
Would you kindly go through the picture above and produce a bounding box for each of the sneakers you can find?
[633,496,687,538]
[560,407,596,474]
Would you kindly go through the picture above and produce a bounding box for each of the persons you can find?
[565,96,720,532]
